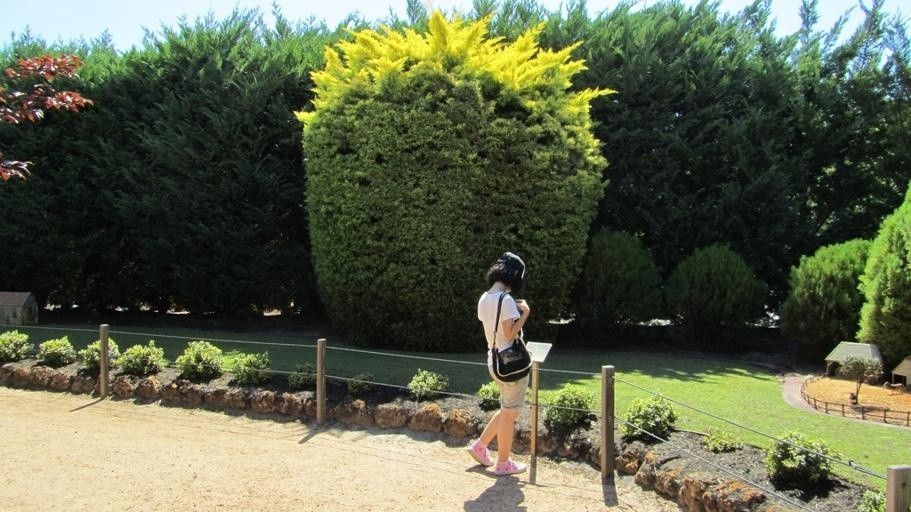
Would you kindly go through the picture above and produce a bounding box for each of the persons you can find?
[465,250,533,477]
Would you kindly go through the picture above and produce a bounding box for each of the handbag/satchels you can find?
[491,291,532,383]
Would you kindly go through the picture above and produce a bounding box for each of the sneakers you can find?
[494,458,527,476]
[468,443,495,467]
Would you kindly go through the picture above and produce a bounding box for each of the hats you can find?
[495,251,527,281]
